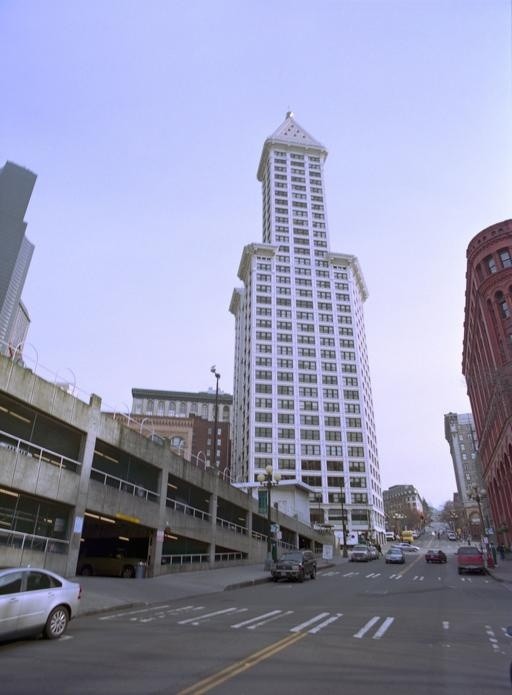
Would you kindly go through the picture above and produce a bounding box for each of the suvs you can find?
[269,549,320,581]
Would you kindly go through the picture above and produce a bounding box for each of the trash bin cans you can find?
[134,561,147,579]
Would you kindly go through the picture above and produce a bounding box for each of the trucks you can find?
[453,545,486,575]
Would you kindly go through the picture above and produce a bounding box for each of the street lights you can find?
[337,473,361,558]
[257,464,282,572]
[392,513,403,530]
[465,481,490,541]
[450,511,458,532]
[208,363,223,467]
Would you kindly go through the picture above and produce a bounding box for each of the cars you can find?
[0,561,83,643]
[349,527,458,566]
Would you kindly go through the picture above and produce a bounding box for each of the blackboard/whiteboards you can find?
[323,544,333,560]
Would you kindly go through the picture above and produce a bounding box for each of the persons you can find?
[500,547,506,561]
[492,548,497,564]
